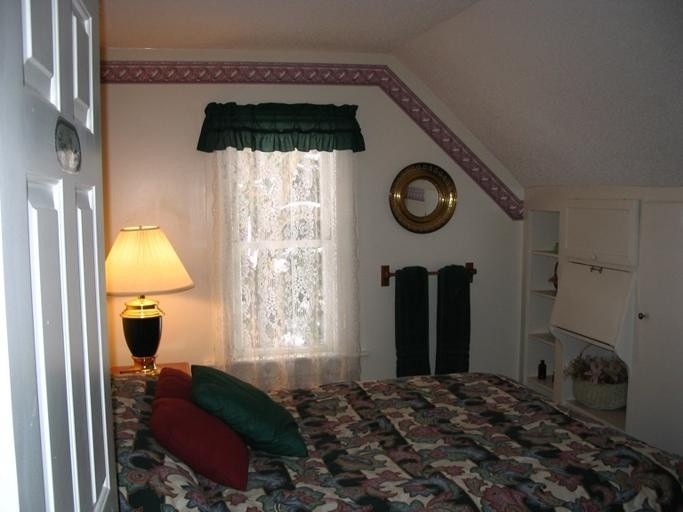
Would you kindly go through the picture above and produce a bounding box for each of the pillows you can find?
[150,358,307,492]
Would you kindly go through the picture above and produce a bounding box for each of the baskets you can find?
[572,342,627,410]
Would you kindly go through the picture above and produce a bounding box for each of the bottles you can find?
[537,360,546,379]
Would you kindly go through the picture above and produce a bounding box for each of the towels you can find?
[395,264,470,378]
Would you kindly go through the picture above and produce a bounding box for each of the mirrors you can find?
[387,162,457,235]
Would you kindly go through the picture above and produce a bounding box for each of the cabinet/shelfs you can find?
[523,187,560,404]
[638,187,682,455]
[560,187,638,436]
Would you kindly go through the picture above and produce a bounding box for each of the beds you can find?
[112,372,682,512]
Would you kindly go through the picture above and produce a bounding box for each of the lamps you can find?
[104,225,193,371]
